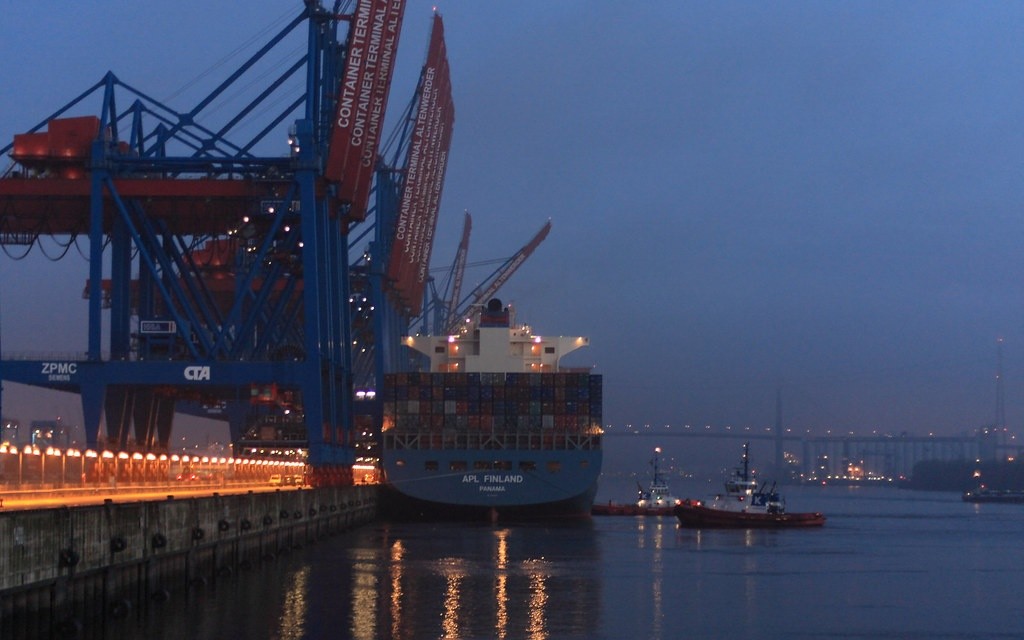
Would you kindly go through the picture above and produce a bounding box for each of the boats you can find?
[672,439,827,530]
[635,451,676,510]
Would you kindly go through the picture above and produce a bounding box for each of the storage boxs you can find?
[383,371,602,448]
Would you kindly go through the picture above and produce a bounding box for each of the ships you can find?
[378,295,608,522]
